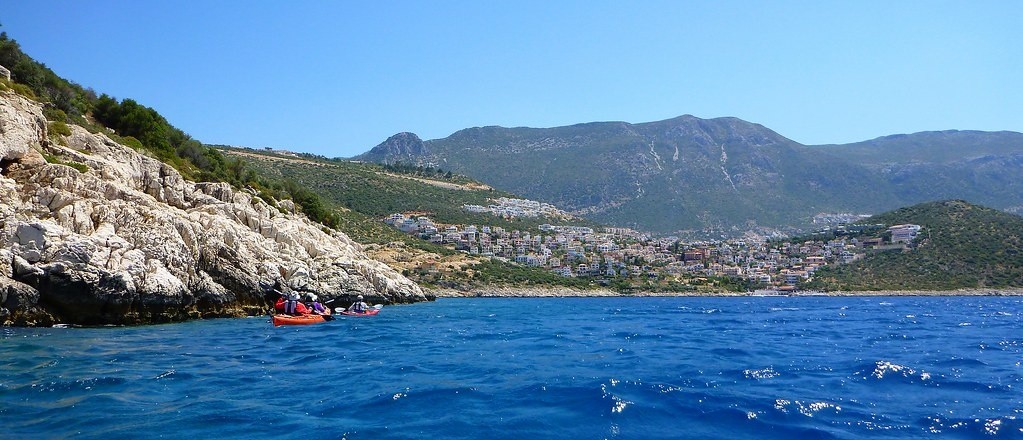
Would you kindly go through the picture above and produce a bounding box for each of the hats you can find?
[357,295,364,300]
[288,291,301,301]
[306,293,318,301]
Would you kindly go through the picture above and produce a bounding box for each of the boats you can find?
[273,307,332,327]
[341,303,384,319]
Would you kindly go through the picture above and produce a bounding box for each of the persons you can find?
[348,294,369,313]
[296,292,325,312]
[274,291,313,315]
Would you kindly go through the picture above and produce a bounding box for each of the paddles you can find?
[325,294,349,303]
[335,304,384,313]
[259,280,285,295]
